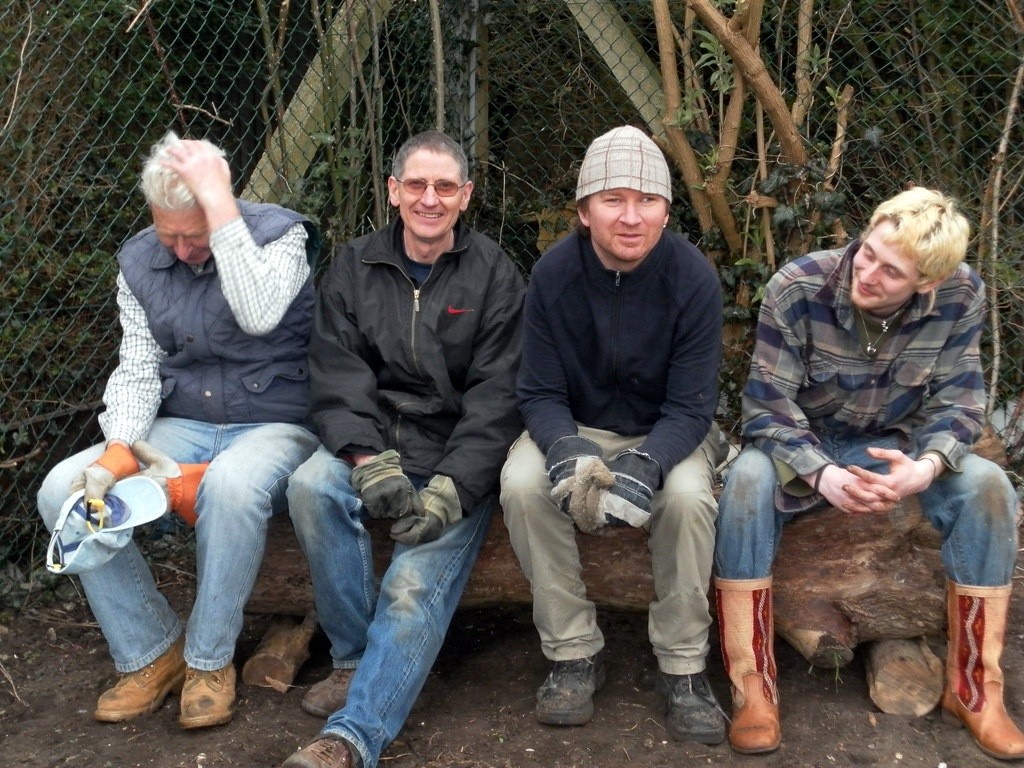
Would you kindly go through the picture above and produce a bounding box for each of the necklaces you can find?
[859,308,902,357]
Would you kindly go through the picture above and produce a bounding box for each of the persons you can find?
[285,130,526,768]
[36,130,321,731]
[499,125,725,745]
[712,186,1024,758]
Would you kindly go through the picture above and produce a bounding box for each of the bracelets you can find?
[814,463,833,494]
[915,458,936,488]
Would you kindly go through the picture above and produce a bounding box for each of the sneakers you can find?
[300,669,353,716]
[657,670,724,744]
[282,738,350,768]
[94,639,186,723]
[535,656,605,726]
[178,663,237,727]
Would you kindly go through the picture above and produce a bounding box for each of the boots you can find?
[941,582,1024,761]
[714,575,782,755]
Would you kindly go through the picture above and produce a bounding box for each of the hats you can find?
[576,125,673,204]
[45,474,168,575]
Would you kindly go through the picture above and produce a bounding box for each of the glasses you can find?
[395,178,467,198]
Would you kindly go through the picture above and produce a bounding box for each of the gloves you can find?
[125,438,206,525]
[350,447,426,520]
[74,441,142,513]
[390,474,463,546]
